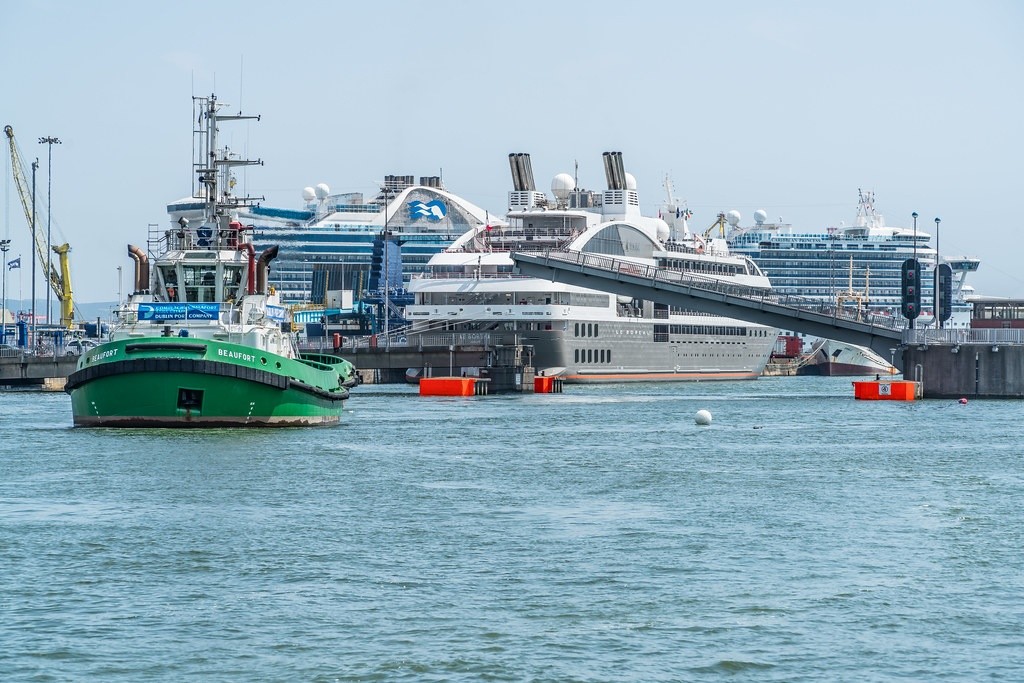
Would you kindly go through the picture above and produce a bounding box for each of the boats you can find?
[721,186,980,351]
[168,167,500,342]
[404,209,779,383]
[795,254,901,376]
[64,89,359,435]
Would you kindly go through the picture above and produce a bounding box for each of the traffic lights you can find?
[901,259,920,319]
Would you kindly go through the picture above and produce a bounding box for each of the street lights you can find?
[911,213,918,329]
[39,135,61,325]
[829,234,837,316]
[934,218,941,330]
[31,156,40,353]
[0,239,11,344]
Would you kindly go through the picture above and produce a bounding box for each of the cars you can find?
[65,338,102,356]
[0,344,31,359]
